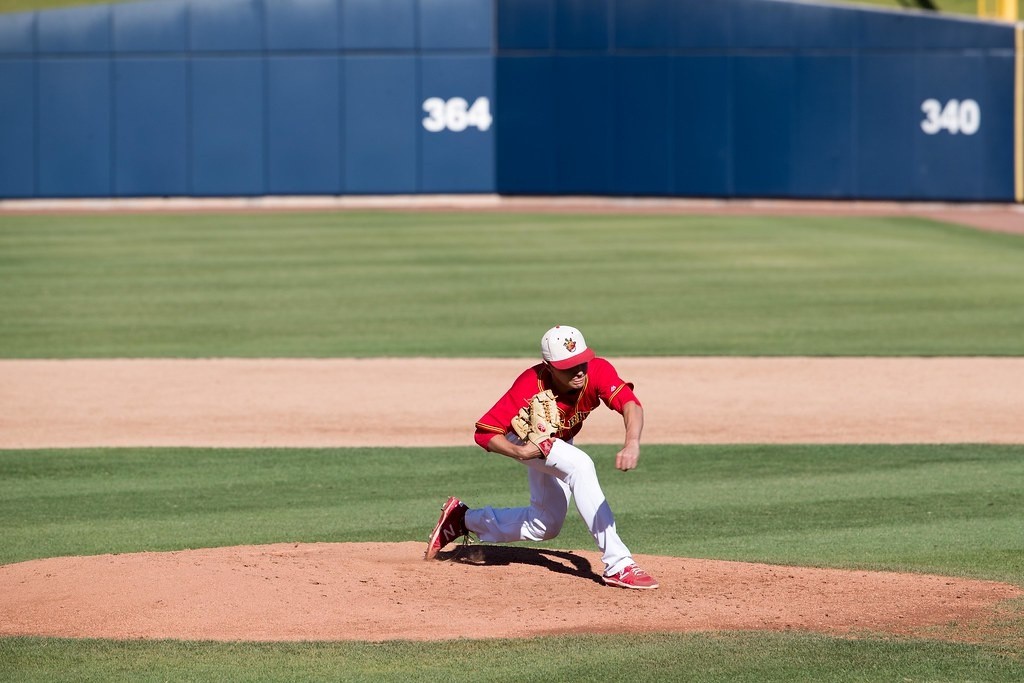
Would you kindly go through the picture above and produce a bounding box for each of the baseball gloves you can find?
[510,388,561,459]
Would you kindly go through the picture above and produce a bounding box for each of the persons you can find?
[422,323,660,589]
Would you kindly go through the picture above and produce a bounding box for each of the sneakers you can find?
[601,564,659,589]
[424,496,475,562]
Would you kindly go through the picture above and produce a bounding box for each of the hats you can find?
[541,325,595,370]
